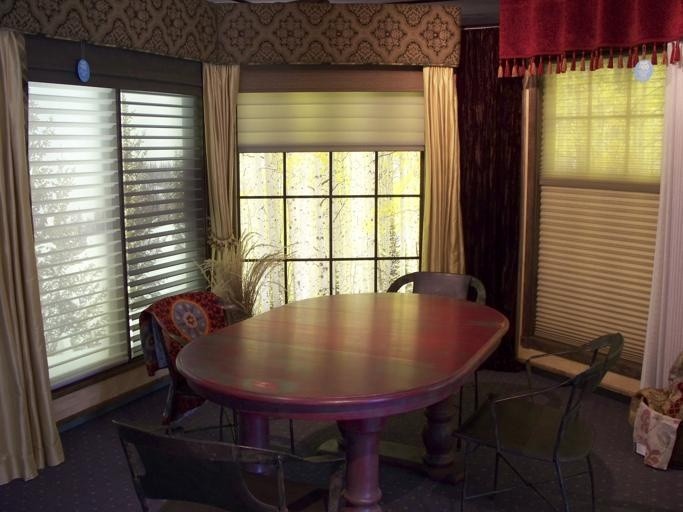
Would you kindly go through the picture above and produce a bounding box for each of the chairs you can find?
[387,272,486,451]
[112,419,346,512]
[430,333,624,512]
[152,292,295,453]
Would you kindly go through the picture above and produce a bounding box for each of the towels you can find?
[632,402,679,471]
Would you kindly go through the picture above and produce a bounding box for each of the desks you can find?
[176,293,509,512]
